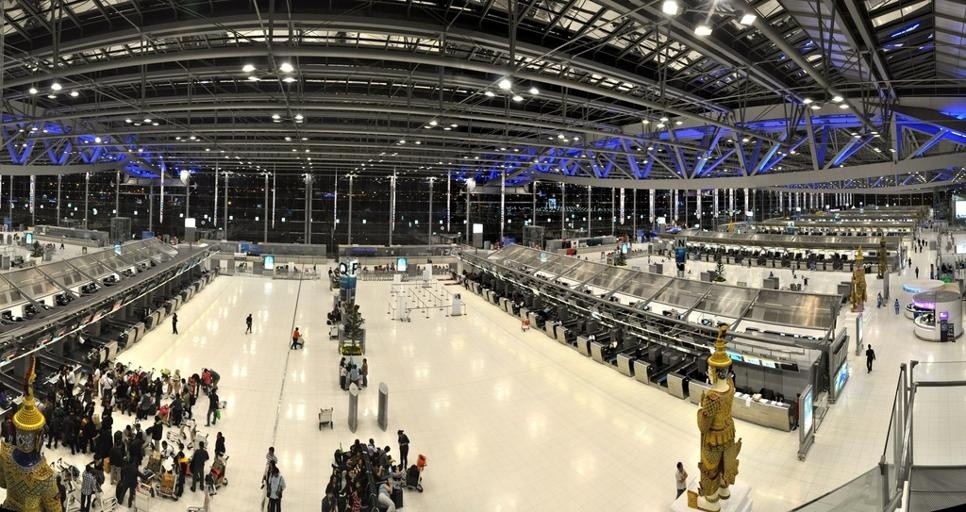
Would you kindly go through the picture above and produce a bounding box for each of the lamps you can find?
[27,78,80,101]
[662,0,758,38]
[241,60,299,85]
[802,88,850,112]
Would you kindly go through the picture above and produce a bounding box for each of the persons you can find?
[877,293,883,308]
[915,266,920,278]
[397,429,410,471]
[675,461,689,499]
[863,262,868,273]
[0,355,64,512]
[244,313,253,334]
[339,357,369,390]
[260,447,286,512]
[864,344,877,375]
[170,311,179,336]
[0,363,226,512]
[866,262,873,272]
[687,324,740,512]
[908,237,927,268]
[290,327,303,351]
[322,438,405,512]
[33,240,38,255]
[894,299,900,314]
[58,237,64,250]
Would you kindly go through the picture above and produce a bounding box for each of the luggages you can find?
[103,456,111,473]
[116,480,124,498]
[160,474,175,495]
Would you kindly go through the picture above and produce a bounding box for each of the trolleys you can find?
[319,408,334,431]
[291,337,304,349]
[329,326,339,340]
[51,417,229,512]
[520,324,530,331]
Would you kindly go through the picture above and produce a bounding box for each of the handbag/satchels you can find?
[214,409,221,419]
